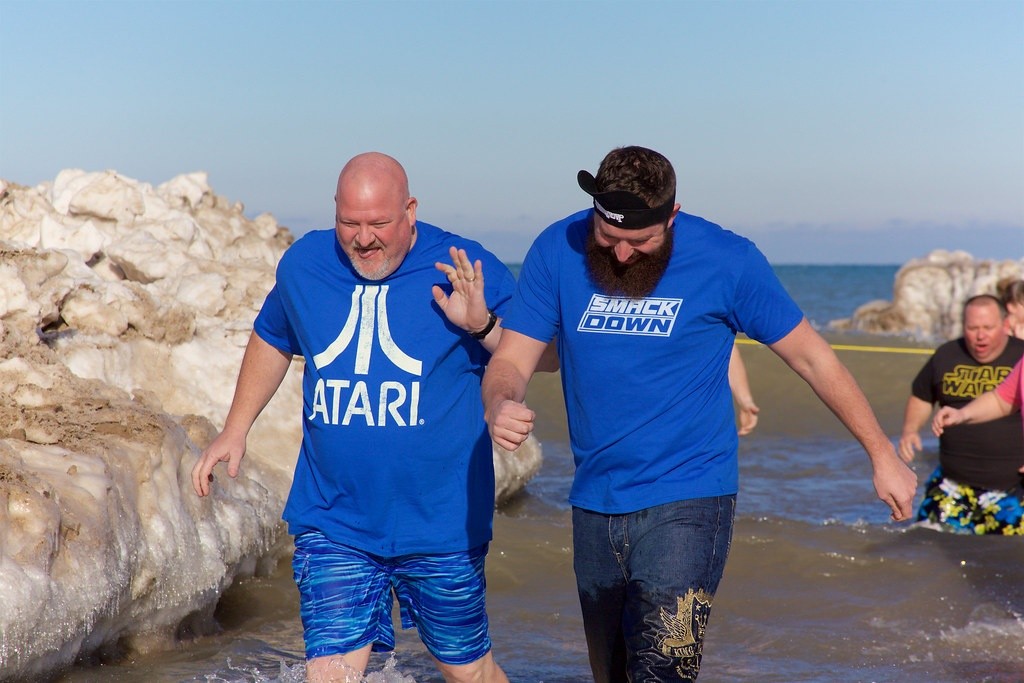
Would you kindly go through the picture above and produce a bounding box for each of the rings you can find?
[466,276,476,283]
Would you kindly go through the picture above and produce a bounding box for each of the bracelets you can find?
[467,309,497,341]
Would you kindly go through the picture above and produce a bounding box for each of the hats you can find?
[576,170,677,229]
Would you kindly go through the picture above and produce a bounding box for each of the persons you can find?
[189,152,565,683]
[897,276,1024,537]
[725,340,761,438]
[477,146,921,683]
